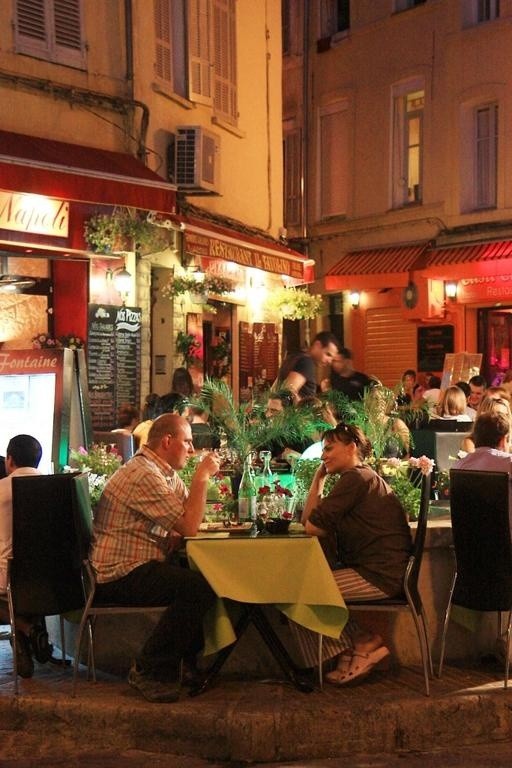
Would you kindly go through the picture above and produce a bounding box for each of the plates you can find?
[197,521,253,532]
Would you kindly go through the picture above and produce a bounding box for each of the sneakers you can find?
[10,630,34,677]
[177,657,220,689]
[33,624,54,663]
[122,657,180,702]
[497,633,512,670]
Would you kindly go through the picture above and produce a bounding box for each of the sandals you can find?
[325,654,352,683]
[338,646,389,683]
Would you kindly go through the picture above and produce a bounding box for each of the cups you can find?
[285,498,299,521]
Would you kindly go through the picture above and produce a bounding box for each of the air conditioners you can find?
[172,122,222,195]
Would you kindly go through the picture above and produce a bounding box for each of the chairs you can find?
[0,471,93,696]
[70,472,186,700]
[316,472,434,696]
[437,467,511,690]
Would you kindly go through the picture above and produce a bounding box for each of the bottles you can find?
[238,462,257,522]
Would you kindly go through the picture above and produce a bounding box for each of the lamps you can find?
[348,290,361,312]
[444,282,457,303]
[182,264,208,286]
[107,264,133,296]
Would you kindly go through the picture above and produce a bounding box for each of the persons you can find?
[111,403,139,433]
[0,434,53,677]
[419,376,441,407]
[172,368,193,396]
[329,348,370,401]
[462,397,511,453]
[143,394,161,420]
[288,422,412,688]
[270,331,340,407]
[87,413,220,704]
[456,382,477,419]
[434,385,470,421]
[265,390,292,419]
[402,370,422,403]
[132,393,190,447]
[450,415,512,472]
[469,376,487,410]
[424,373,433,389]
[190,397,220,449]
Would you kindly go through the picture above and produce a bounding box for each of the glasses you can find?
[173,397,189,412]
[337,422,359,448]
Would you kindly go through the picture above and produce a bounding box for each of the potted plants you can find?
[178,375,315,520]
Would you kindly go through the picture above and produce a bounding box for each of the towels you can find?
[185,531,230,539]
